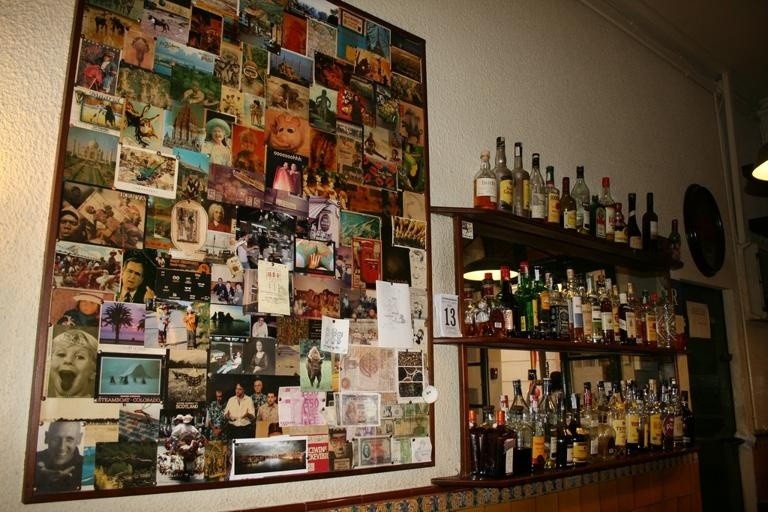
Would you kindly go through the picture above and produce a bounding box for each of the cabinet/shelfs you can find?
[430,201,703,485]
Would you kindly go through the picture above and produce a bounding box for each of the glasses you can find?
[243,67,258,79]
[329,437,348,447]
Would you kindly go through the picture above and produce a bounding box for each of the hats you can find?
[73,291,106,307]
[331,7,338,13]
[205,117,232,138]
[182,413,194,424]
[173,413,184,421]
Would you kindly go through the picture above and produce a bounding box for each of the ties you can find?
[123,292,132,303]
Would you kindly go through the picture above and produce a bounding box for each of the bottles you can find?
[492,138,511,210]
[570,166,590,233]
[512,143,531,216]
[545,166,561,228]
[642,192,658,252]
[466,371,693,479]
[601,176,615,241]
[628,193,642,250]
[473,150,499,210]
[614,202,629,242]
[463,261,675,346]
[590,194,606,240]
[530,153,546,221]
[669,219,682,259]
[561,176,577,230]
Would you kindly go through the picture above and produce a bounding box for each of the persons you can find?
[295,287,340,318]
[208,389,229,440]
[389,149,402,164]
[249,380,267,411]
[202,89,218,111]
[279,162,301,176]
[58,291,103,327]
[249,339,268,375]
[203,118,231,167]
[216,351,241,374]
[252,318,268,337]
[234,282,243,305]
[342,294,349,313]
[329,427,353,473]
[346,94,366,124]
[49,329,98,398]
[413,302,423,319]
[414,329,424,345]
[224,383,254,439]
[353,294,377,319]
[315,90,331,123]
[117,258,154,304]
[183,306,198,350]
[364,132,386,160]
[250,99,263,128]
[208,204,232,233]
[59,209,84,241]
[223,93,242,121]
[212,278,225,296]
[156,304,171,347]
[33,422,84,493]
[101,50,117,92]
[257,392,279,422]
[181,80,203,105]
[161,414,201,449]
[55,251,120,291]
[234,229,291,270]
[306,252,320,269]
[225,283,233,301]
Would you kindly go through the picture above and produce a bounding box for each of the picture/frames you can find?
[20,0,436,505]
